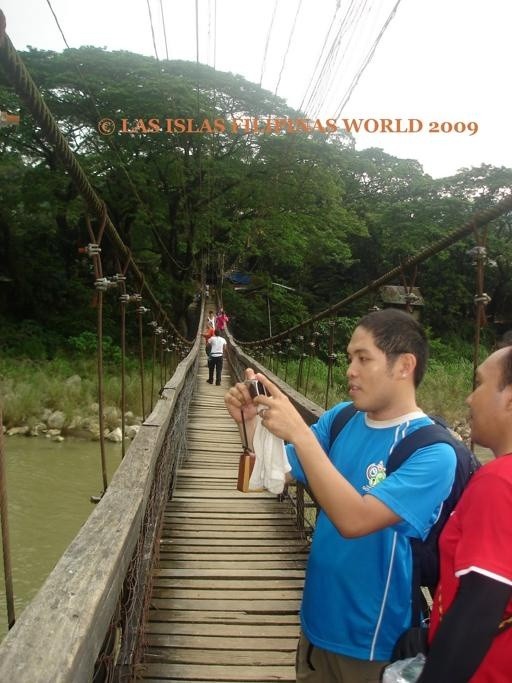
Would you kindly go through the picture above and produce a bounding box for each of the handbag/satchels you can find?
[206,347,211,356]
[391,627,427,663]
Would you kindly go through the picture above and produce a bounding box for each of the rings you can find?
[262,408,266,417]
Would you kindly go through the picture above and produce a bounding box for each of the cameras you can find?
[243,376,269,404]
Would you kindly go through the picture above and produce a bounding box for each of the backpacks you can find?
[387,424,482,587]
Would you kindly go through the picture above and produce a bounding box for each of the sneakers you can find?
[207,380,221,385]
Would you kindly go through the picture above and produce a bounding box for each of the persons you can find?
[387,331,512,682]
[203,281,231,387]
[222,306,460,682]
